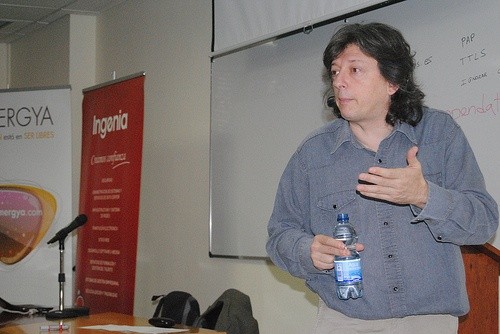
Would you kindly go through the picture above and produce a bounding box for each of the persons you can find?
[265,22,500,334]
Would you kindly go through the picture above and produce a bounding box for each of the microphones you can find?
[47,214,88,244]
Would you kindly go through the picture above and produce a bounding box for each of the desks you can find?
[0,311,229,334]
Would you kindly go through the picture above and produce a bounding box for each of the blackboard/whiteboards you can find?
[207,1,500,261]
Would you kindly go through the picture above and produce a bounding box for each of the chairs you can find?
[153,289,259,334]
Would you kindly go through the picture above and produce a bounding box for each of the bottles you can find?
[333,213,363,300]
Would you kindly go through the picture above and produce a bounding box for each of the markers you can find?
[39,324,69,331]
